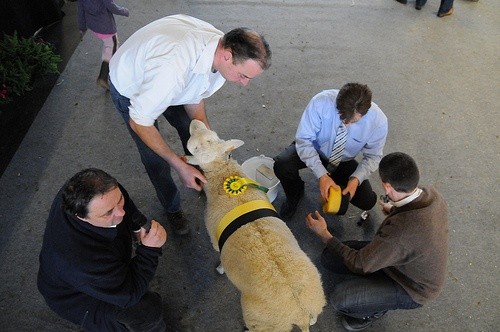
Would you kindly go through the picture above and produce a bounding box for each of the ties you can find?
[326,124,348,173]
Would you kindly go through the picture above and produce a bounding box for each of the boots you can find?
[96,61,112,91]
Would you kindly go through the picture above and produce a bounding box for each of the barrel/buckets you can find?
[241,155,281,202]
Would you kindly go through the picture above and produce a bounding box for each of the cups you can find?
[129,215,151,233]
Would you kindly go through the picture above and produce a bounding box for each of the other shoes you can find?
[341,310,388,331]
[437,7,454,17]
[396,0,407,4]
[167,211,189,235]
[416,1,425,10]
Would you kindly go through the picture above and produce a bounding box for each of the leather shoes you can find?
[280,200,300,218]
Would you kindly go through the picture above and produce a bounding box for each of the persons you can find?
[36,167,168,332]
[77,0,130,90]
[108,12,272,236]
[305,152,450,332]
[272,83,388,218]
[396,0,479,17]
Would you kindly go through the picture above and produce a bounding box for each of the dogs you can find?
[179,119,327,332]
